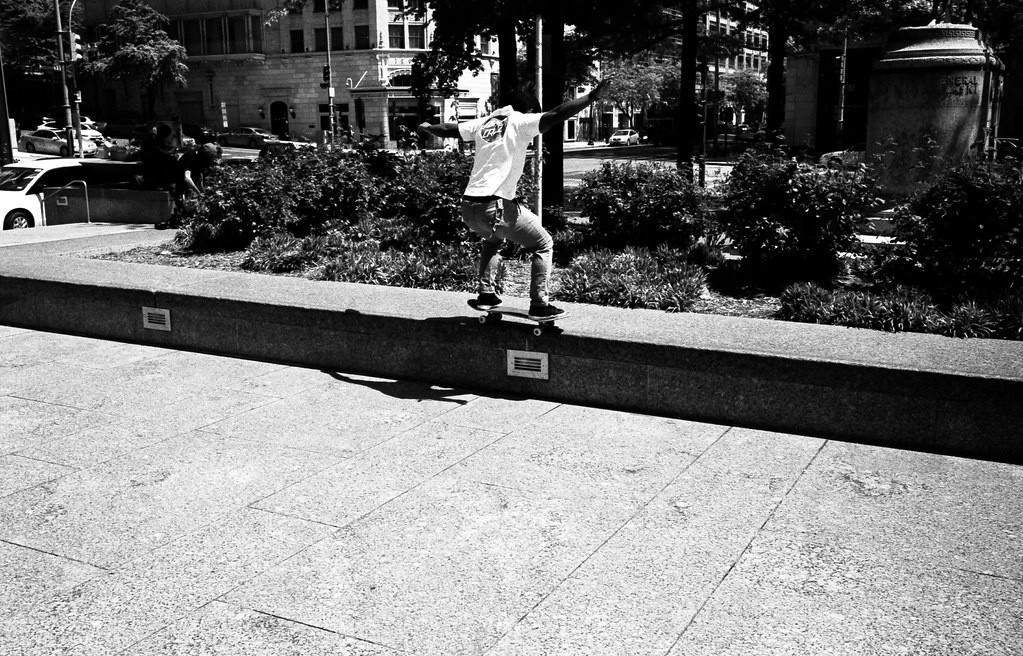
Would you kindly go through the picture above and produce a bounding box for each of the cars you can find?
[737,124,750,131]
[609,129,641,145]
[37,114,104,146]
[20,127,98,157]
[182,122,213,142]
[42,117,99,129]
[215,126,281,146]
[819,144,866,173]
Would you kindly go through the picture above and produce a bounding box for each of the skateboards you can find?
[469,298,570,333]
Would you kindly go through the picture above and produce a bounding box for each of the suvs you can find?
[0,158,173,241]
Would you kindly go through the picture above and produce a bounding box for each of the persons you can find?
[140,124,223,229]
[419,77,612,317]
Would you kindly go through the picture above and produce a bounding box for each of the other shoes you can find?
[529,302,566,319]
[476,293,503,310]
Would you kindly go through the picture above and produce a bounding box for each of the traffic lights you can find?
[323,64,331,81]
[63,31,84,62]
